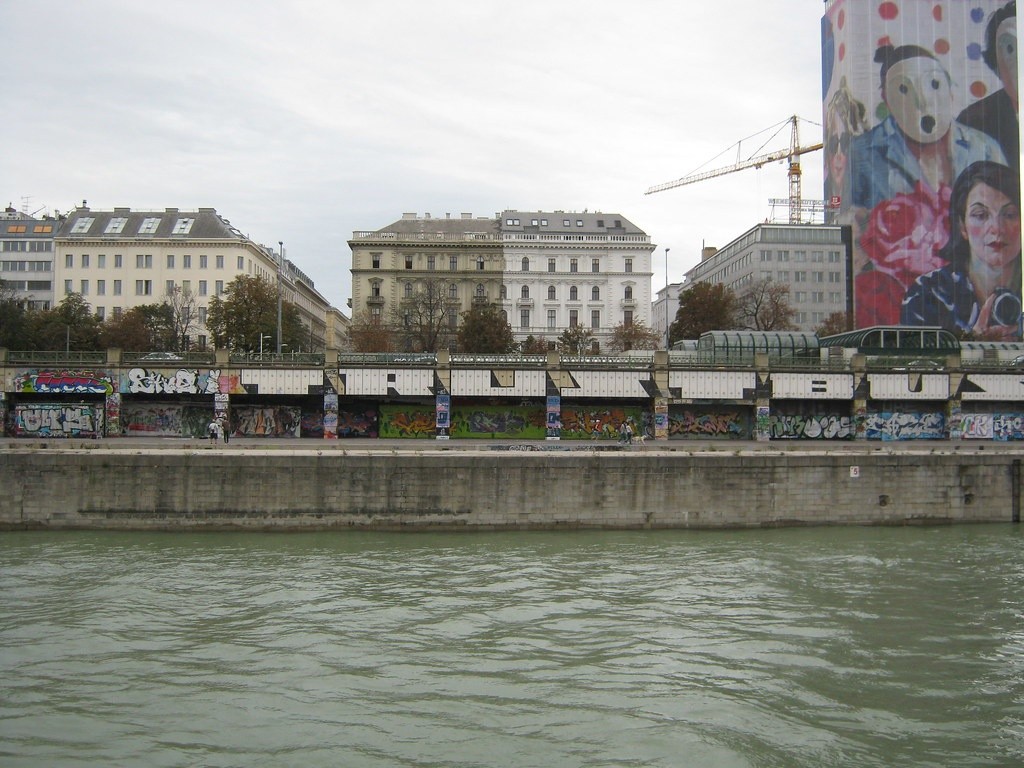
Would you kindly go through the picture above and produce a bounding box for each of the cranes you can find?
[643,115,826,225]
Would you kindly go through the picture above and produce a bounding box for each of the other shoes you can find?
[617,441,621,445]
[225,443,230,445]
[625,442,629,444]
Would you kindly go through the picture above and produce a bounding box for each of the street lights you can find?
[259,333,273,367]
[664,247,670,353]
[275,241,284,362]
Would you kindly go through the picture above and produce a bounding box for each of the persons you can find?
[222,418,231,443]
[594,420,600,443]
[208,419,220,444]
[618,421,633,445]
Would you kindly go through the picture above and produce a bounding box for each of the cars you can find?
[891,357,944,371]
[140,352,184,362]
[1006,355,1024,368]
